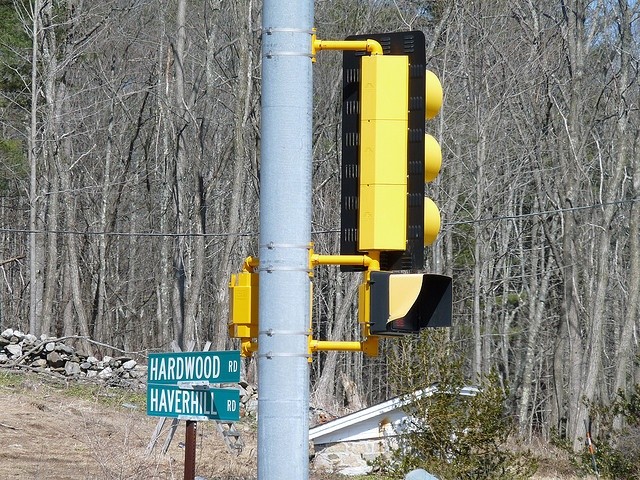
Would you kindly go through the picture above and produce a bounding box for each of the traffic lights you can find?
[370,271,452,334]
[340,30,443,272]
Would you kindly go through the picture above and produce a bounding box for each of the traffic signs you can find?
[146,384,240,421]
[148,350,240,384]
